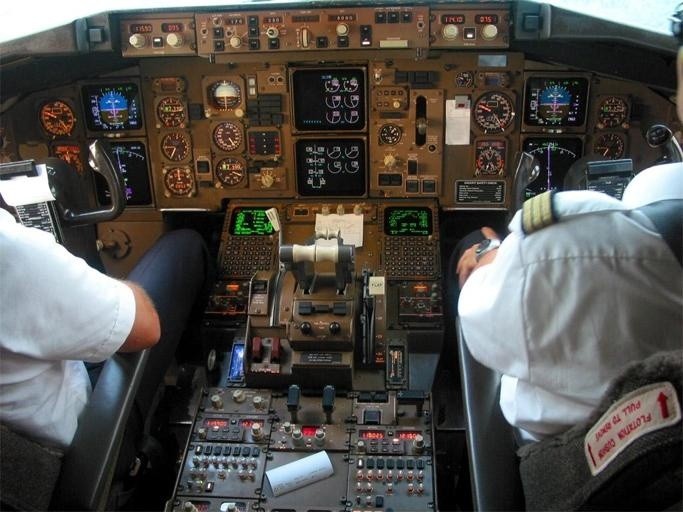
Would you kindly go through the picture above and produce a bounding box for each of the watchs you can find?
[475,239,502,262]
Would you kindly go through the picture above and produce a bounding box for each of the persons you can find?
[441,2,683,457]
[1,207,219,479]
